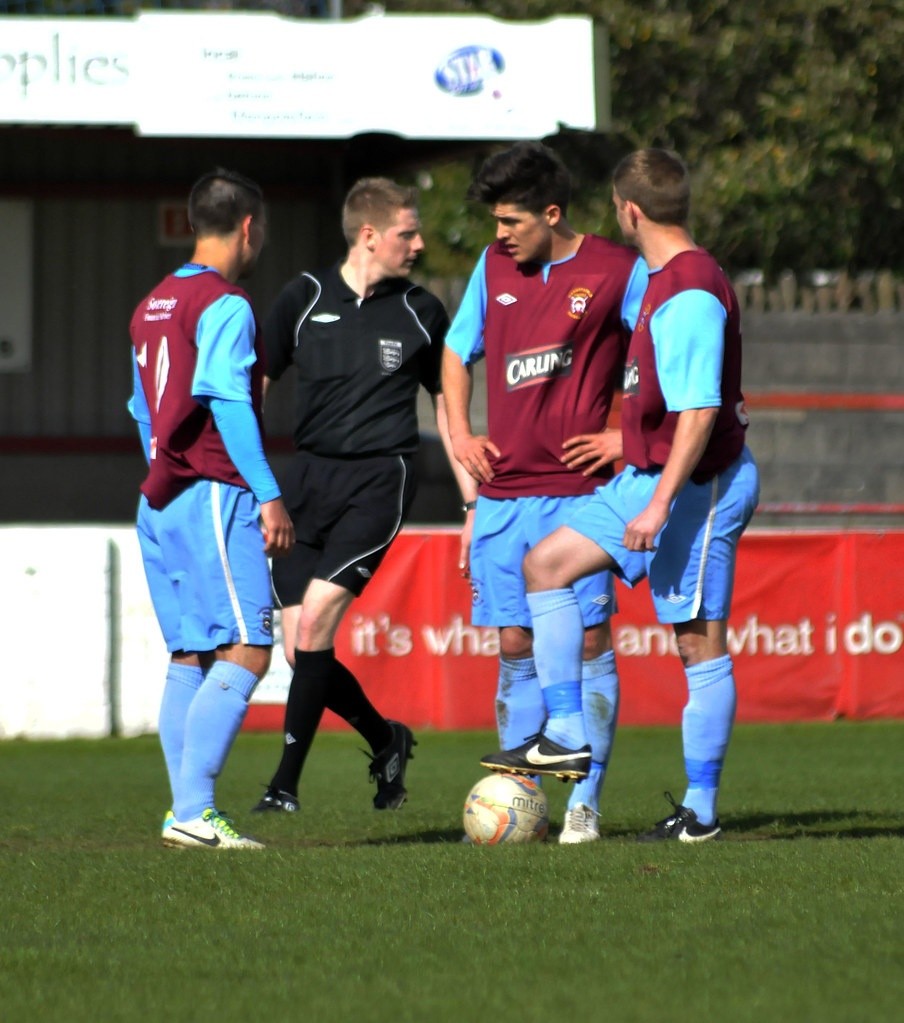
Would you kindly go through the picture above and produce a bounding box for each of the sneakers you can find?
[559,803,603,845]
[358,720,417,811]
[480,734,593,783]
[631,792,721,845]
[251,783,300,814]
[161,807,266,851]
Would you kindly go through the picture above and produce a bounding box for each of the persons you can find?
[440,142,648,845]
[129,174,298,851]
[481,149,761,843]
[253,177,479,815]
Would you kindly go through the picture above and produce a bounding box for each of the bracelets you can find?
[461,500,477,512]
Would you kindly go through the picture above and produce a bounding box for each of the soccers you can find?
[461,771,552,845]
